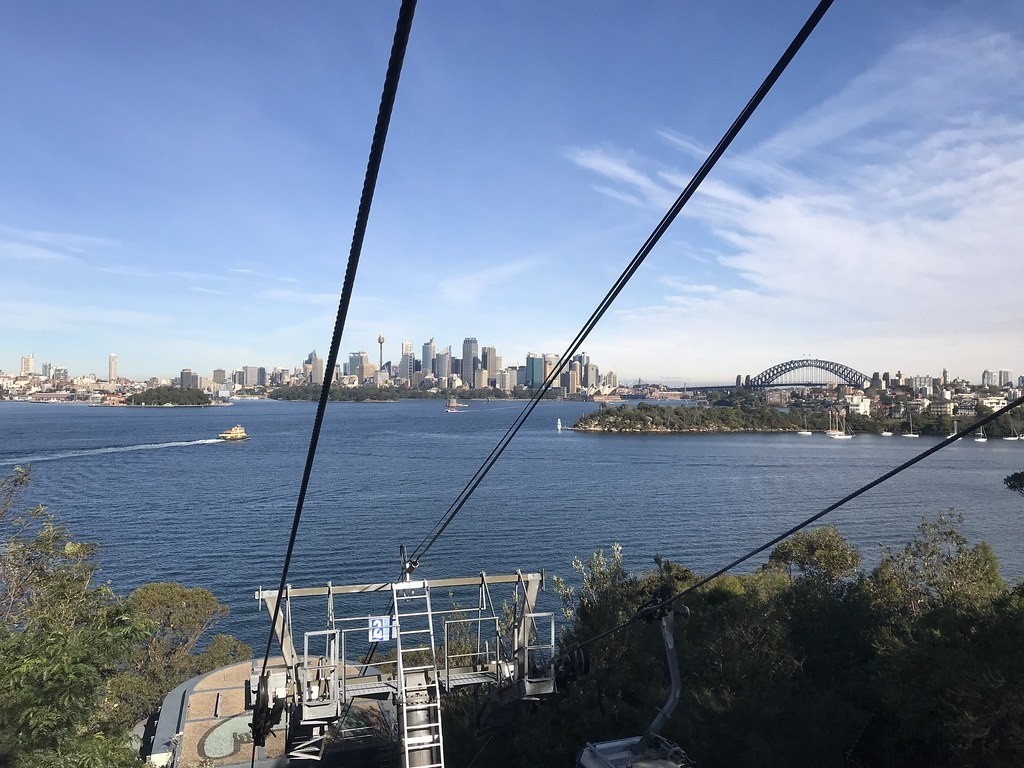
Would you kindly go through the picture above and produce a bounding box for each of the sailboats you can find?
[797,410,1024,443]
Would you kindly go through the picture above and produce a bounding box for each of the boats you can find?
[219,424,251,441]
[444,407,457,413]
[446,398,463,407]
